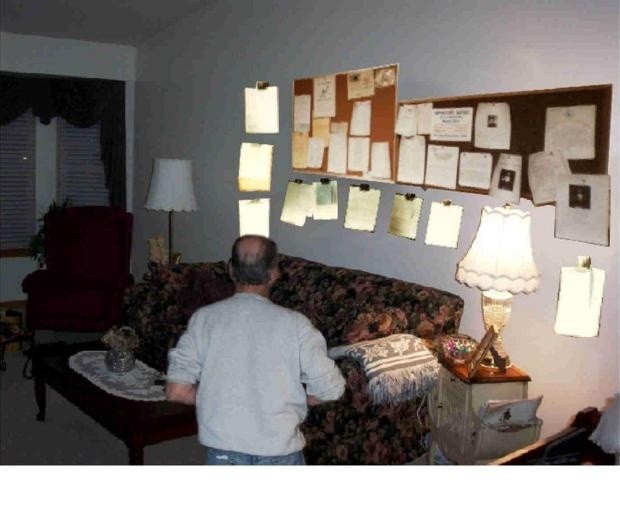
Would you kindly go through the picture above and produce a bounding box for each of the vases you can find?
[105,349,137,375]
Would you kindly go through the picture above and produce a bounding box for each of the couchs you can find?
[121,240,465,463]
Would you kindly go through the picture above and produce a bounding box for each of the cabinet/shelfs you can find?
[429,352,535,464]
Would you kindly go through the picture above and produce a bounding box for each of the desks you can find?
[27,338,199,464]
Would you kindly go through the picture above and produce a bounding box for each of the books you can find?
[478,396,545,424]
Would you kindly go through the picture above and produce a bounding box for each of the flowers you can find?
[97,321,145,353]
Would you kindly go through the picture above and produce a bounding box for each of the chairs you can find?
[20,197,140,385]
[488,387,620,464]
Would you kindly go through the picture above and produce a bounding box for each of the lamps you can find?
[452,201,544,371]
[144,157,200,264]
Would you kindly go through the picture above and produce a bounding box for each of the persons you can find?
[165,236,347,465]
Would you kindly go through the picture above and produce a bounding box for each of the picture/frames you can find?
[464,323,500,377]
